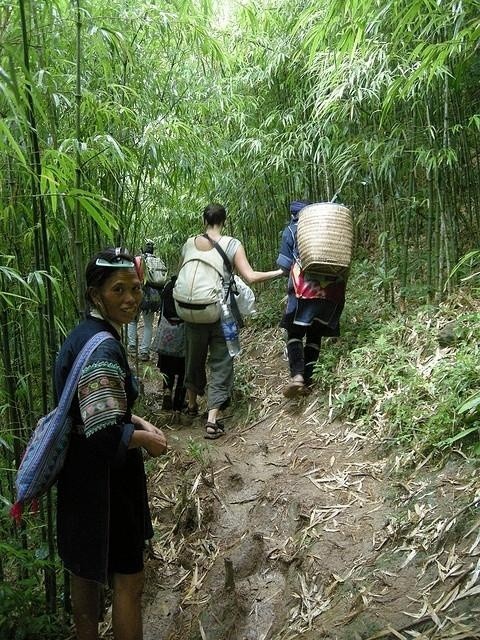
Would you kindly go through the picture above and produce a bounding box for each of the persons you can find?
[156,275,189,411]
[52,246,168,640]
[127,239,155,361]
[176,202,285,440]
[276,199,347,399]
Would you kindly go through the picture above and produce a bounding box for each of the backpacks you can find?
[162,275,185,326]
[171,248,226,324]
[142,255,169,289]
[139,286,162,315]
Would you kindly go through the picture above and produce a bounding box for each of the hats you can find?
[141,239,154,253]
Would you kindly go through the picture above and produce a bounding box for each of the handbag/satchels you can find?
[11,408,73,524]
[229,273,257,316]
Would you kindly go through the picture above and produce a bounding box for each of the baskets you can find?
[295,202,355,278]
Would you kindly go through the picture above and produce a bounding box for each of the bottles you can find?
[221,303,242,357]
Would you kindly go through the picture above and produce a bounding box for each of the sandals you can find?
[203,421,226,440]
[182,406,199,419]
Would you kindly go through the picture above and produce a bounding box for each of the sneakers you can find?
[282,378,305,398]
[141,353,150,361]
[161,396,172,411]
[127,344,137,354]
[173,398,189,411]
